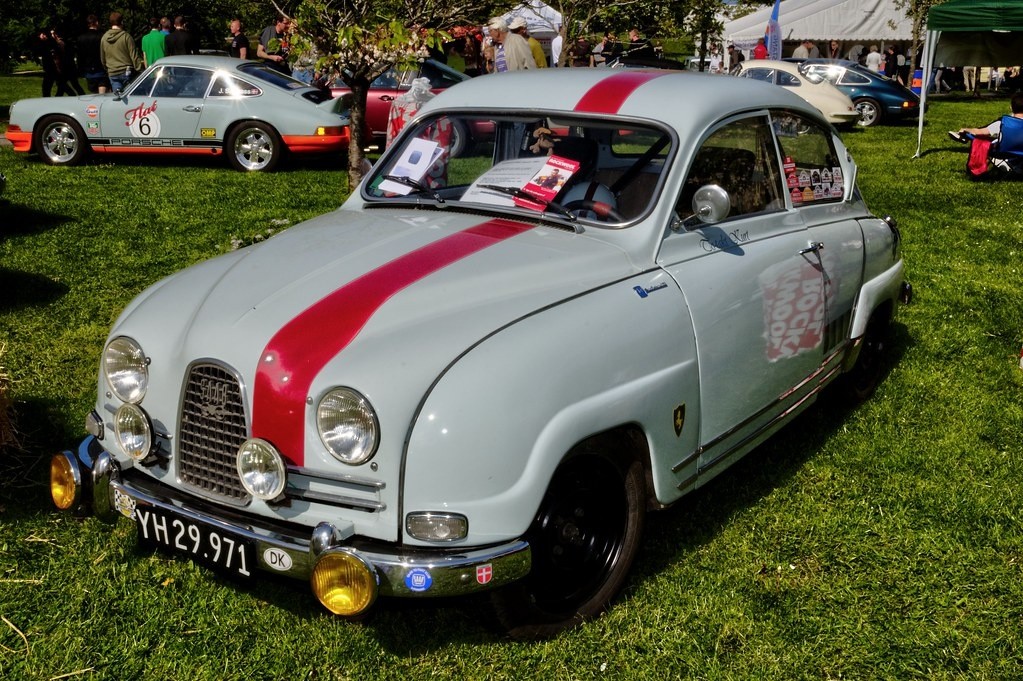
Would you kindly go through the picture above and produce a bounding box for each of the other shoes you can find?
[948,131,968,144]
[947,88,952,94]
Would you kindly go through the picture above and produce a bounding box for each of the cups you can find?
[99,86,106,93]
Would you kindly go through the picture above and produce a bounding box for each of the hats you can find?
[507,16,527,30]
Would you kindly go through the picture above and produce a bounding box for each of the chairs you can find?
[963,115,1023,184]
[543,136,599,184]
[677,146,757,220]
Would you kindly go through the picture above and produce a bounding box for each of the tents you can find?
[498,0,587,69]
[911,0,1023,159]
[722,0,941,93]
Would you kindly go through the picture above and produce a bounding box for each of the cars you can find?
[53,66,914,635]
[288,55,498,159]
[4,54,356,172]
[782,57,932,123]
[728,62,860,127]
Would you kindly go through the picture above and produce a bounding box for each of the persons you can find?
[727,39,1023,156]
[38,10,295,100]
[481,17,660,161]
